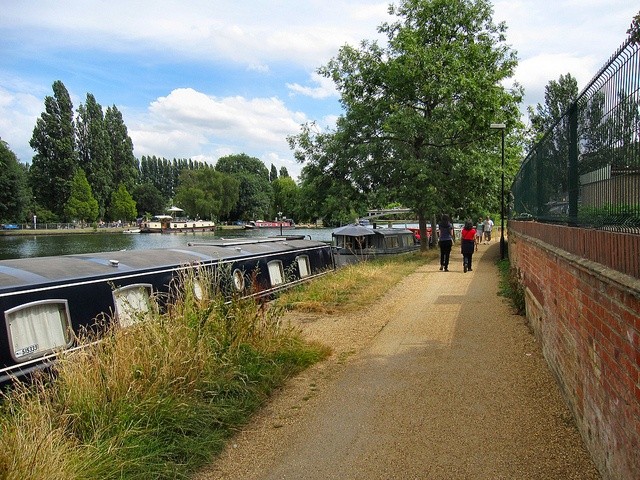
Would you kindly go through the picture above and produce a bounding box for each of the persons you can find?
[438,214,454,271]
[477,218,484,244]
[484,216,494,245]
[461,220,478,273]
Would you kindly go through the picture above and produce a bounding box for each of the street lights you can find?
[34,196,36,215]
[489,124,506,260]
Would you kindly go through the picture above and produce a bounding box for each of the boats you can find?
[268,234,305,240]
[331,225,421,268]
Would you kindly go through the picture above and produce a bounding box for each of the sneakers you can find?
[439,265,443,270]
[464,264,467,273]
[445,269,449,272]
[468,269,472,271]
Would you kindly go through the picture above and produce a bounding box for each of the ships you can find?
[140,219,215,232]
[246,211,295,228]
[0,238,336,372]
